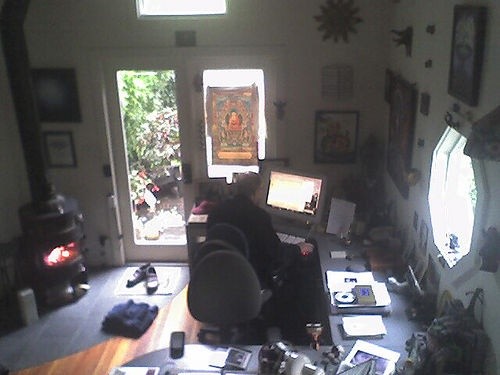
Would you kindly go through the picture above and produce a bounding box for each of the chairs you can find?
[188,222,272,345]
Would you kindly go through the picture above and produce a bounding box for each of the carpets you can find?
[116,266,183,296]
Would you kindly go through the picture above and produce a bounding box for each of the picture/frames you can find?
[447,4,487,105]
[42,131,77,168]
[313,110,359,165]
[31,67,82,123]
[386,75,417,199]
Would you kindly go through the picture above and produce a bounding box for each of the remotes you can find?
[170,331,185,359]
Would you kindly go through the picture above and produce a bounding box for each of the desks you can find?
[120,343,407,375]
[259,201,432,346]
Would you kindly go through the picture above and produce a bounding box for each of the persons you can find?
[206,171,314,345]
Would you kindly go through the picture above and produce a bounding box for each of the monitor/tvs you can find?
[260,166,327,223]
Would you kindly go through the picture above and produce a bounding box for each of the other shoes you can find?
[145,266,158,290]
[127,263,152,287]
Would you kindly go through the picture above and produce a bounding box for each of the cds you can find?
[333,292,355,304]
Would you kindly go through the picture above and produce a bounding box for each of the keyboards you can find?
[275,232,305,246]
